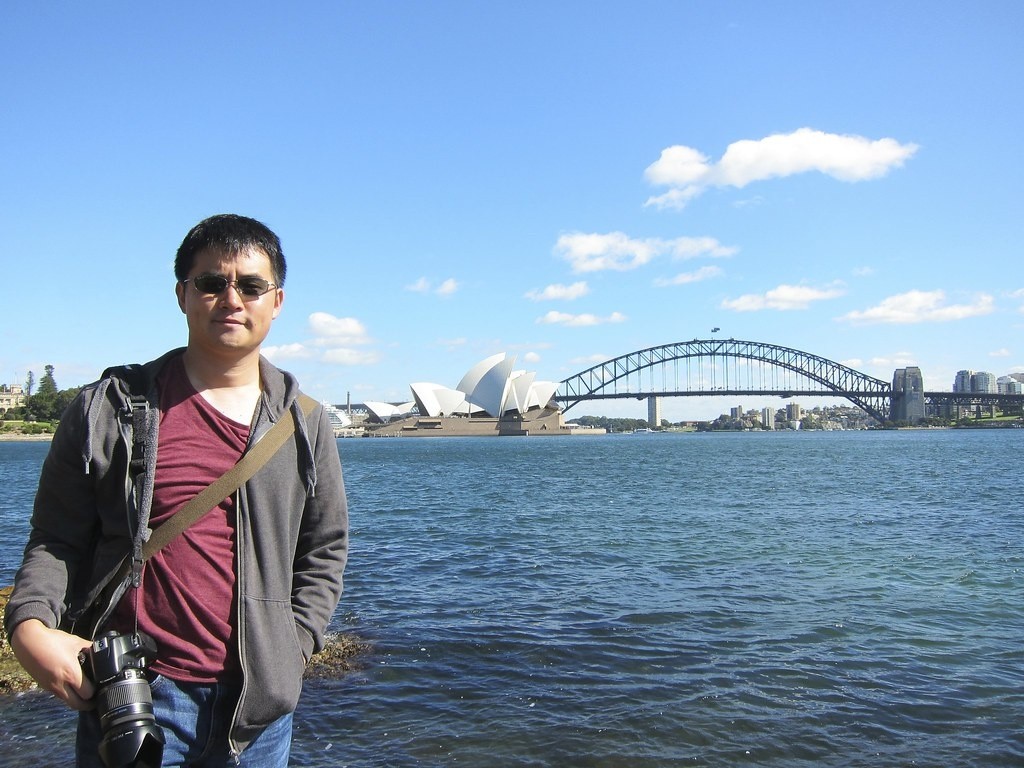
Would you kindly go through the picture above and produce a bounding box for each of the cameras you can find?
[78,631,166,768]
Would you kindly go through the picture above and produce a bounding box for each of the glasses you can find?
[183,275,278,296]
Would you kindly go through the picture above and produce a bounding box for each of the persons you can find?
[4,214,347,768]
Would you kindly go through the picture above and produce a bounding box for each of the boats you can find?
[630,428,659,433]
